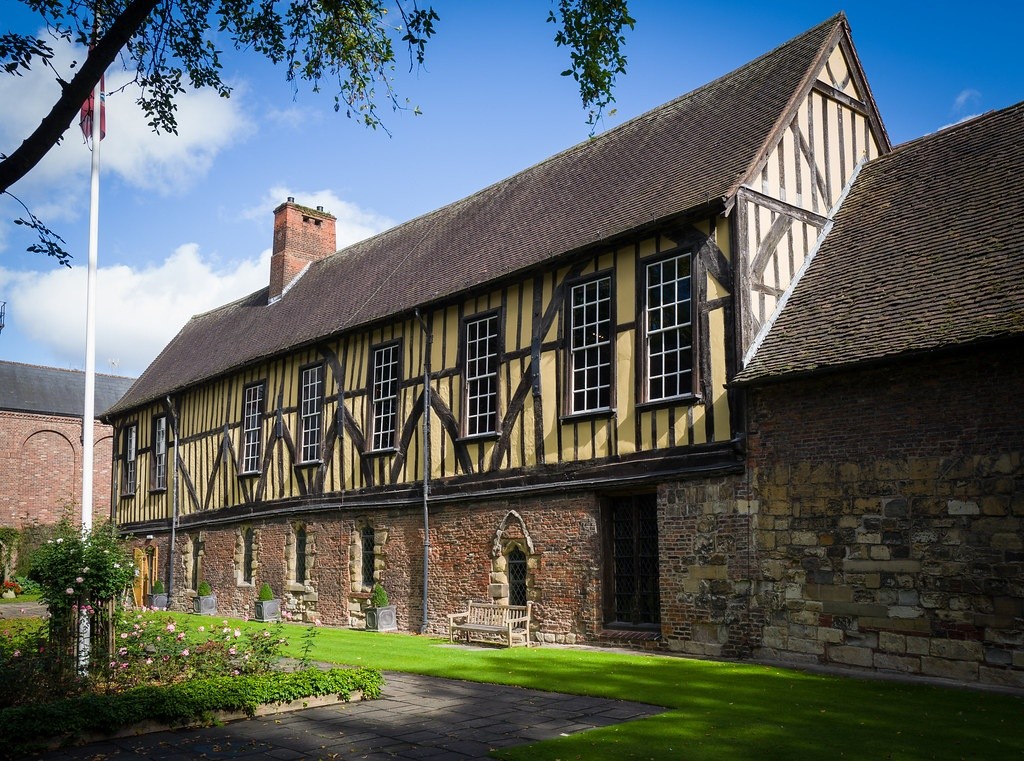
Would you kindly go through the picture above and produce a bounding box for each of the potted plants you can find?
[146,582,167,609]
[365,584,397,633]
[193,581,218,614]
[254,582,282,621]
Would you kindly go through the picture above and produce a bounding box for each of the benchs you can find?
[446,600,532,649]
